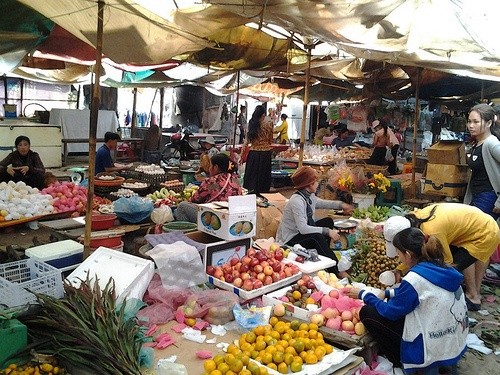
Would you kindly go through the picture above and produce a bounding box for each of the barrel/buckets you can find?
[88,242,124,255]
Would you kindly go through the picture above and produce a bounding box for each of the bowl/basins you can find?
[83,215,118,229]
[77,234,125,247]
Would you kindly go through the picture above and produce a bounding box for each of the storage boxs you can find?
[207,256,303,299]
[185,230,252,273]
[422,163,469,197]
[196,192,257,242]
[24,239,84,269]
[261,279,338,320]
[135,171,185,193]
[65,246,155,305]
[426,141,466,167]
[0,257,64,308]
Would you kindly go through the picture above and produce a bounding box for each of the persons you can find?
[332,128,356,148]
[175,136,243,222]
[0,136,46,191]
[272,114,289,145]
[238,104,274,193]
[369,119,401,175]
[275,166,354,276]
[95,132,130,176]
[382,201,500,310]
[462,102,500,221]
[346,227,470,375]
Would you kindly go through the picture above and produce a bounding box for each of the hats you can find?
[372,119,380,128]
[383,216,411,258]
[290,166,319,188]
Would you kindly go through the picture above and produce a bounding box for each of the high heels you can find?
[465,294,480,310]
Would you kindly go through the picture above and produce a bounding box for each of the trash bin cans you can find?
[180,168,198,184]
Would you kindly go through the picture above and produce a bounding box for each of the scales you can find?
[68,167,89,184]
[330,219,358,250]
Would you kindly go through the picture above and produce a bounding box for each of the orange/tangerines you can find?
[185,318,196,327]
[203,316,334,375]
[0,354,62,375]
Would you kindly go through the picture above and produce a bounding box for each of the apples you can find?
[0,180,53,222]
[177,299,235,325]
[205,248,367,335]
[42,181,94,212]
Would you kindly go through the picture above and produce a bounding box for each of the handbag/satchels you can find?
[385,146,394,162]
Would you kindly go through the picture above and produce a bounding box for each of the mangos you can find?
[183,186,199,200]
[234,222,252,234]
[203,212,220,230]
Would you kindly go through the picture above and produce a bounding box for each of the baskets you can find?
[0,257,64,310]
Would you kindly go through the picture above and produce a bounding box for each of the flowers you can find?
[332,171,392,193]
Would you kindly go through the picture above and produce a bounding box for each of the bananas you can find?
[353,204,390,221]
[148,186,183,203]
